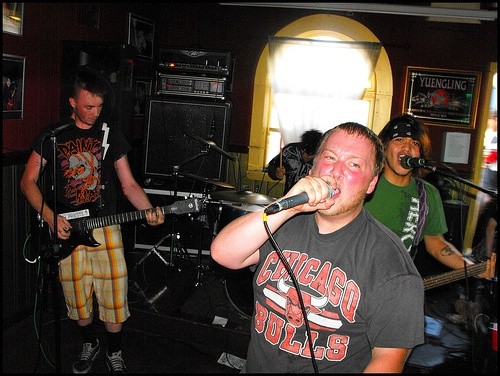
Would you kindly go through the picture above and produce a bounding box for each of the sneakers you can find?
[72,339,100,374]
[104,350,128,376]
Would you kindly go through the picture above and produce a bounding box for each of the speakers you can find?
[142,98,232,184]
[412,199,469,293]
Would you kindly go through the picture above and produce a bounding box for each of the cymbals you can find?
[178,171,236,189]
[210,189,278,205]
[179,129,237,163]
[442,198,471,209]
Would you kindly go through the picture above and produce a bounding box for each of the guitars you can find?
[28,197,205,267]
[422,260,487,291]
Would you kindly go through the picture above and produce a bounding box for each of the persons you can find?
[482,110,497,192]
[211,121,423,374]
[364,113,496,278]
[268,130,323,195]
[20,64,165,373]
[3,77,17,110]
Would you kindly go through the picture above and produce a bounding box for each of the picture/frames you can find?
[2,3,32,37]
[400,65,482,130]
[128,12,155,59]
[2,54,25,120]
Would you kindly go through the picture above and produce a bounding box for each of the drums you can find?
[213,188,280,240]
[224,264,261,322]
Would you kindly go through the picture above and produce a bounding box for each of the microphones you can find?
[264,181,336,216]
[401,155,428,169]
[43,118,75,137]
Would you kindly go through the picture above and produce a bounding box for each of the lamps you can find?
[220,1,498,22]
